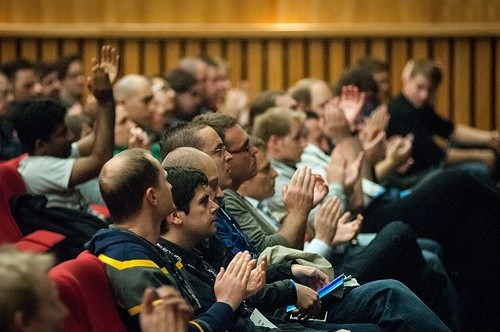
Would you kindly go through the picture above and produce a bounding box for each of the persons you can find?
[0,44,500,332]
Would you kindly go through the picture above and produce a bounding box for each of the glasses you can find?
[187,88,197,97]
[206,146,225,155]
[153,85,172,94]
[228,144,250,154]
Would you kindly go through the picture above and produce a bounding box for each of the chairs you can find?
[0,165,74,264]
[50,258,127,332]
